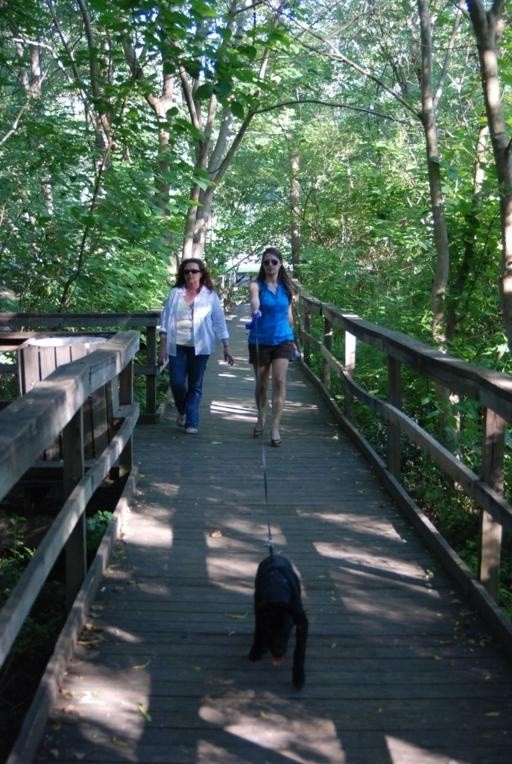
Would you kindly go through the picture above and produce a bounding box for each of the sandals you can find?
[175,414,187,428]
[271,429,282,448]
[253,426,266,440]
[185,425,199,435]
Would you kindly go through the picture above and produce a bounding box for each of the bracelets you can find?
[223,344,230,349]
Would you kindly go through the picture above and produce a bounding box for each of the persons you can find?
[157,257,235,436]
[246,246,303,446]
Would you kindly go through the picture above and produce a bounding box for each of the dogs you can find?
[247,554,309,691]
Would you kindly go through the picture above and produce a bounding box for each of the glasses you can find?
[181,269,201,275]
[262,259,280,267]
